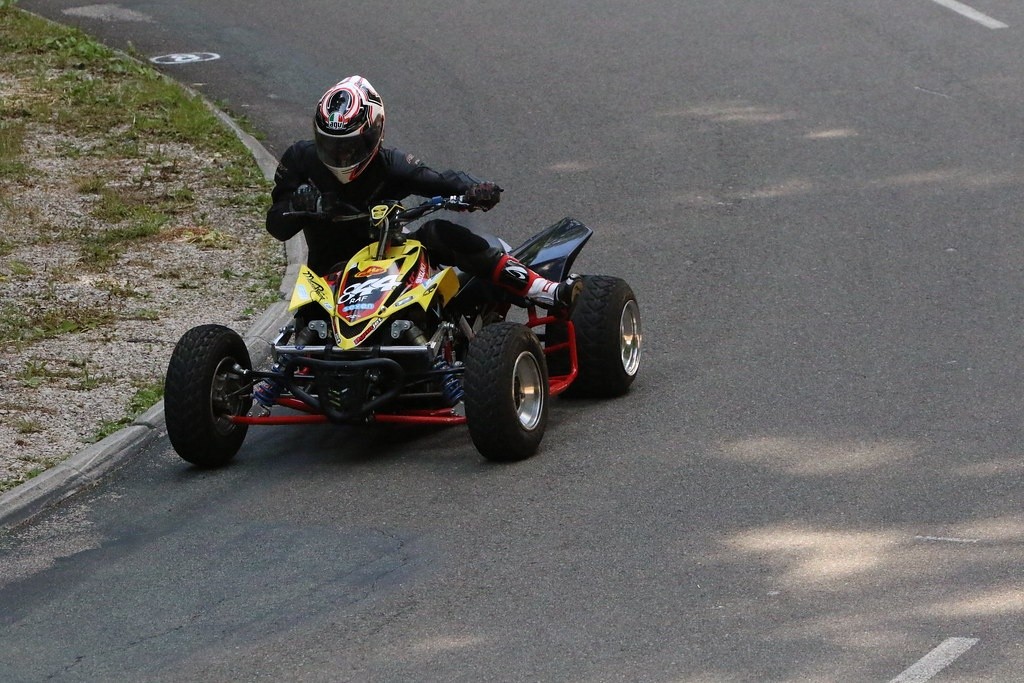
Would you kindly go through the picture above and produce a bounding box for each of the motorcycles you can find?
[164,193,645,469]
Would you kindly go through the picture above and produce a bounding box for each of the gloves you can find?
[290,186,322,214]
[465,181,501,213]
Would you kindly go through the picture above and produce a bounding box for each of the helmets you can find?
[314,75,385,184]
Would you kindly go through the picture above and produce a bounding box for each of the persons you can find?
[265,75,582,375]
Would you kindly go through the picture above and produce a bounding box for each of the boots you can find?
[490,254,583,317]
